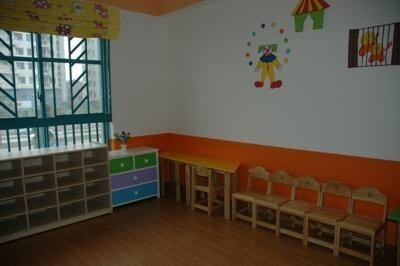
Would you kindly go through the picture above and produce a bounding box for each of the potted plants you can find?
[113,130,130,152]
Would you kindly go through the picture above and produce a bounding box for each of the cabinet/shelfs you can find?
[0,142,112,243]
[107,147,160,208]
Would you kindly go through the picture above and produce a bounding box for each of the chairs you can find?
[231,167,388,266]
[190,165,224,217]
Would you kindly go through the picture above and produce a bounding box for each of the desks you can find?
[185,157,240,220]
[386,205,400,266]
[159,150,201,202]
